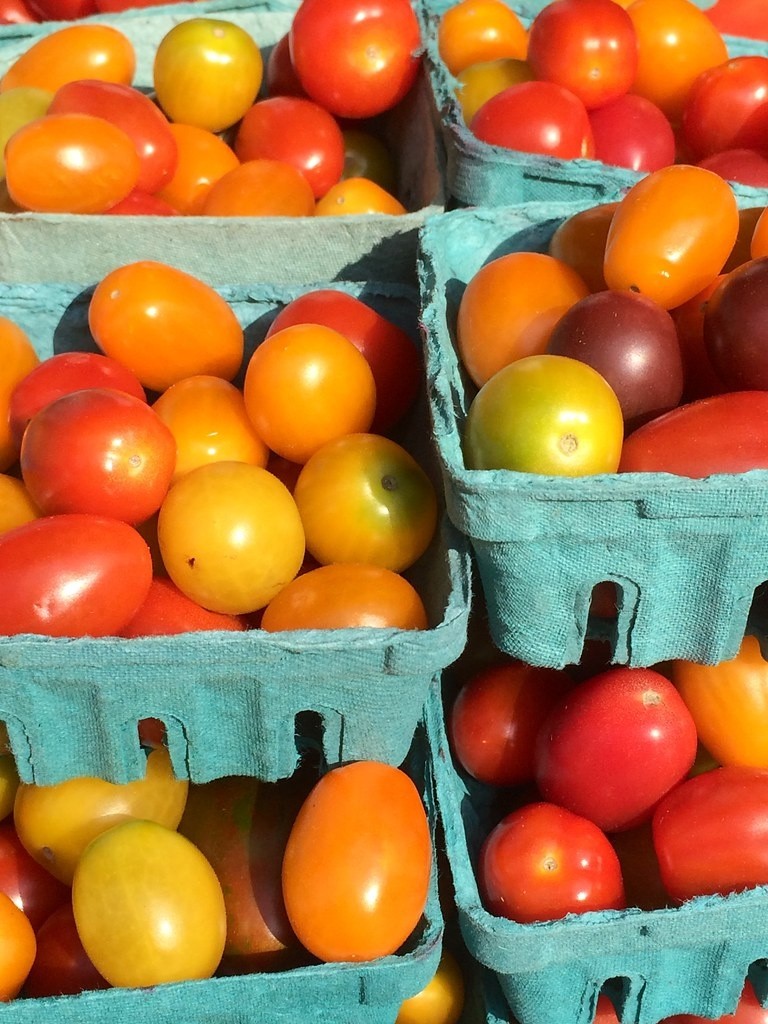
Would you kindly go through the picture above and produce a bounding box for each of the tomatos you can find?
[0,0,767,1024]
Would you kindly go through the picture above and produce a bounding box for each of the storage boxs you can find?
[0,0,767,1024]
